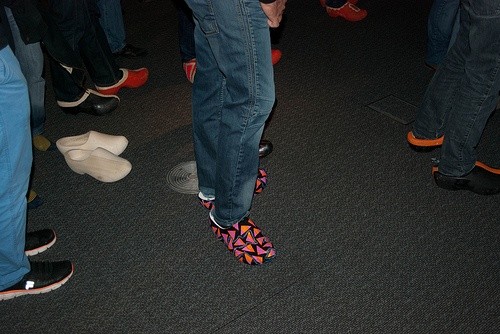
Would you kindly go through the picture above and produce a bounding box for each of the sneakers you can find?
[0,259,73,301]
[24,228,56,256]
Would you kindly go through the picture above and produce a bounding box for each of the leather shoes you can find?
[112,44,148,59]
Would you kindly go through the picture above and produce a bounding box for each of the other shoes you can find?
[64,147,132,182]
[95,66,149,95]
[182,58,198,84]
[431,160,500,195]
[56,130,128,156]
[406,130,444,153]
[57,88,120,116]
[258,140,273,158]
[198,168,267,210]
[271,49,283,65]
[208,208,277,266]
[320,0,358,8]
[326,2,367,22]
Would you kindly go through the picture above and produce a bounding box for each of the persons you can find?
[406,0,500,193]
[1,1,151,300]
[178,0,283,86]
[319,0,369,21]
[187,0,287,264]
[427,0,461,70]
